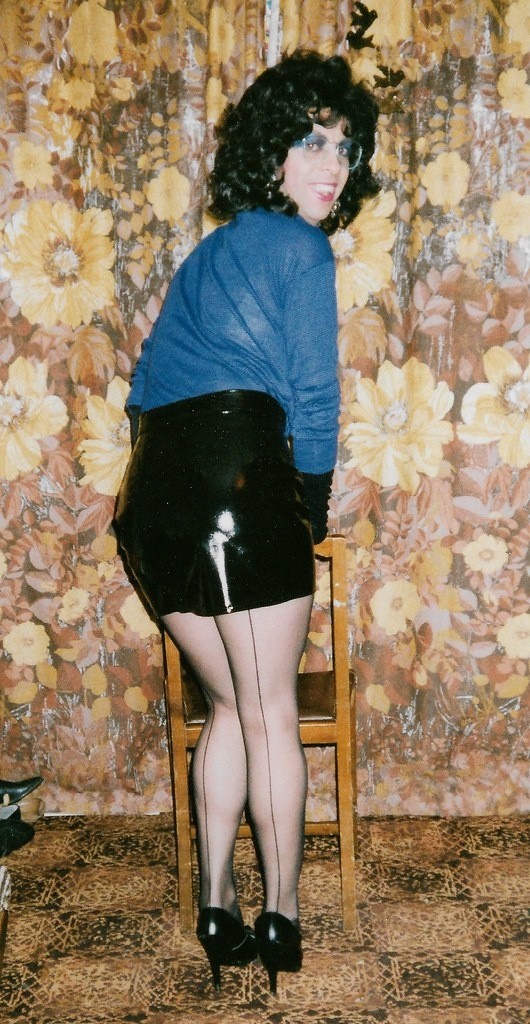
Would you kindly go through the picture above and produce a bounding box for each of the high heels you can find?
[196,907,258,993]
[254,913,302,995]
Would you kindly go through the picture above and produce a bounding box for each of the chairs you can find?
[164,531,357,930]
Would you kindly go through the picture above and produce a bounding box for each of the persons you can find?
[113,50,381,997]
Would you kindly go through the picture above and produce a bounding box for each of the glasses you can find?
[294,136,362,169]
